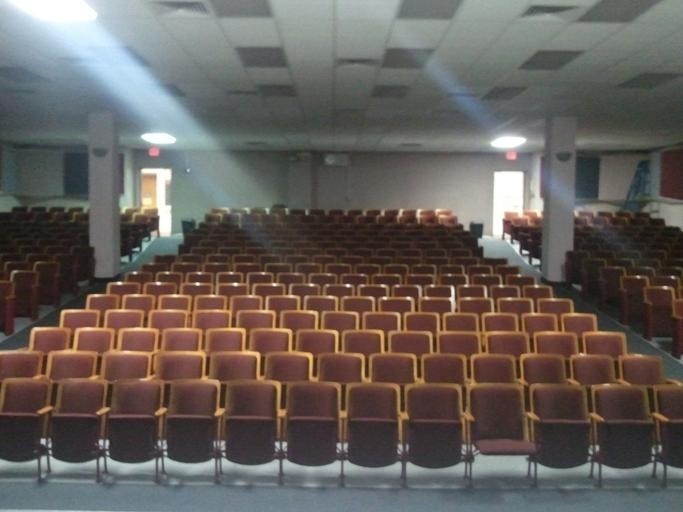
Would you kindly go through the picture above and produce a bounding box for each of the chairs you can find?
[0,202,683,489]
